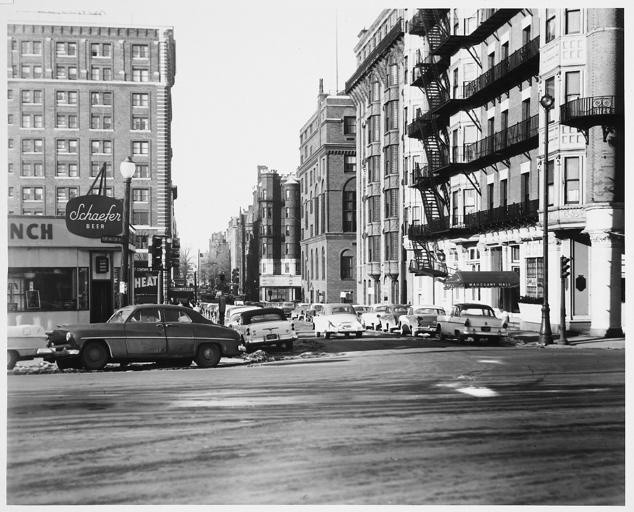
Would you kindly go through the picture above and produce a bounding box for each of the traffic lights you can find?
[165,238,179,271]
[148,233,163,270]
[560,257,571,280]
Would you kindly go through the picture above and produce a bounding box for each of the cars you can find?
[361,302,510,342]
[199,299,297,354]
[291,302,372,338]
[7,323,49,368]
[44,303,240,368]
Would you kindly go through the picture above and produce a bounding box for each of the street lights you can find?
[539,95,552,346]
[193,266,198,301]
[120,157,135,309]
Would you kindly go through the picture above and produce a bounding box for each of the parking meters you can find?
[218,296,226,325]
